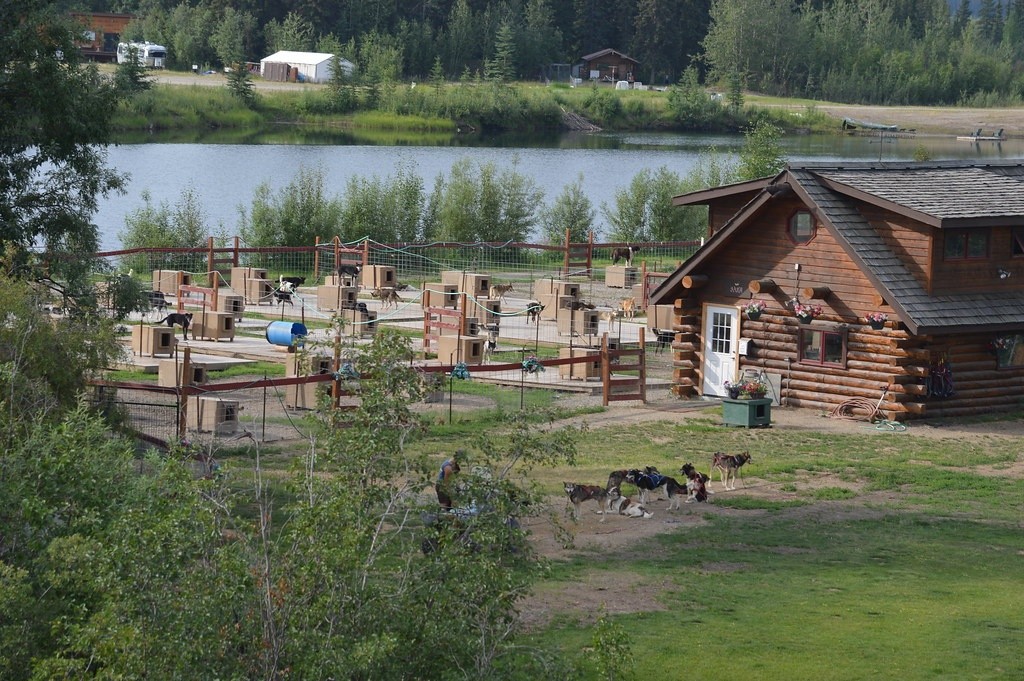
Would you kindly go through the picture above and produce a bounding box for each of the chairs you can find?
[652,327,675,355]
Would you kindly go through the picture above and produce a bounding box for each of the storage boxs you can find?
[439,334,483,364]
[647,303,674,333]
[605,265,638,289]
[459,273,493,298]
[100,262,397,436]
[578,335,619,364]
[421,282,458,309]
[557,308,599,337]
[442,270,477,286]
[554,282,580,302]
[722,396,774,427]
[438,315,479,336]
[559,346,601,381]
[541,293,576,319]
[466,299,501,326]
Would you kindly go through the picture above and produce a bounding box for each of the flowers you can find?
[743,380,769,392]
[863,311,889,323]
[723,380,745,390]
[742,298,767,312]
[794,300,822,318]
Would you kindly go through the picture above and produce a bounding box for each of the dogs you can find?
[482,340,497,365]
[154,312,194,341]
[348,302,369,316]
[334,262,363,288]
[618,296,636,322]
[489,282,514,305]
[272,274,307,310]
[563,300,619,334]
[140,290,172,321]
[610,245,641,268]
[651,327,686,357]
[525,301,546,326]
[370,281,408,311]
[563,450,753,523]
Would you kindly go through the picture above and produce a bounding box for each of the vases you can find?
[747,310,761,319]
[870,319,885,329]
[749,390,766,398]
[728,389,739,398]
[799,314,813,324]
